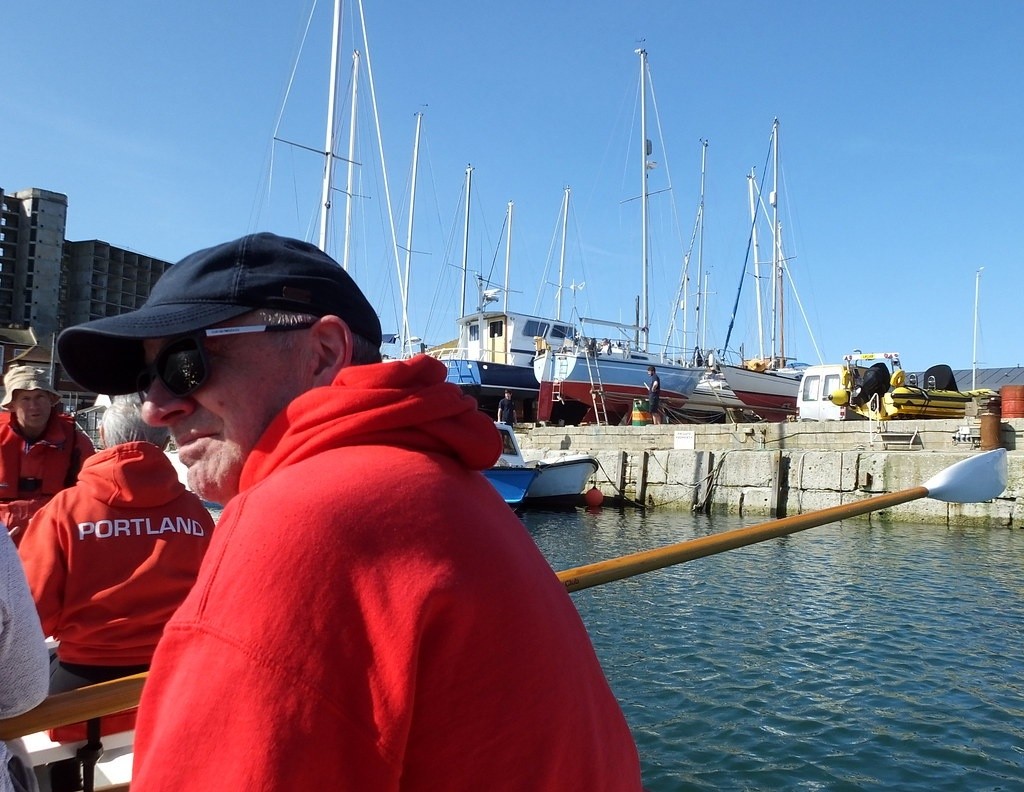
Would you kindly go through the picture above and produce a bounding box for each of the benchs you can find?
[4,729,135,767]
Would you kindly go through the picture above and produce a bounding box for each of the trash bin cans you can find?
[981,413,1001,450]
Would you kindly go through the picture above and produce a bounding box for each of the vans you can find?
[795,364,883,420]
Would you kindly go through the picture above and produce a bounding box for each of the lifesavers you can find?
[889,369,906,388]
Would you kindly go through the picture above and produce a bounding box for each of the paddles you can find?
[1,446,1011,748]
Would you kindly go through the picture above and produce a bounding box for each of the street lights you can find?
[971,266,986,394]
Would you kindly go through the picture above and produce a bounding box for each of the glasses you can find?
[134,323,314,399]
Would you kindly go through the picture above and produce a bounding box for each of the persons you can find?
[0,366,216,792]
[497,389,517,429]
[0,522,51,792]
[56,232,646,792]
[648,366,661,425]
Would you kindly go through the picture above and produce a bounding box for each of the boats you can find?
[477,420,602,514]
[828,352,1003,421]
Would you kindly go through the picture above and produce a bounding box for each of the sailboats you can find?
[268,0,826,428]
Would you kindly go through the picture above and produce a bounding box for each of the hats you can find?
[504,390,512,393]
[0,366,62,410]
[55,232,382,397]
